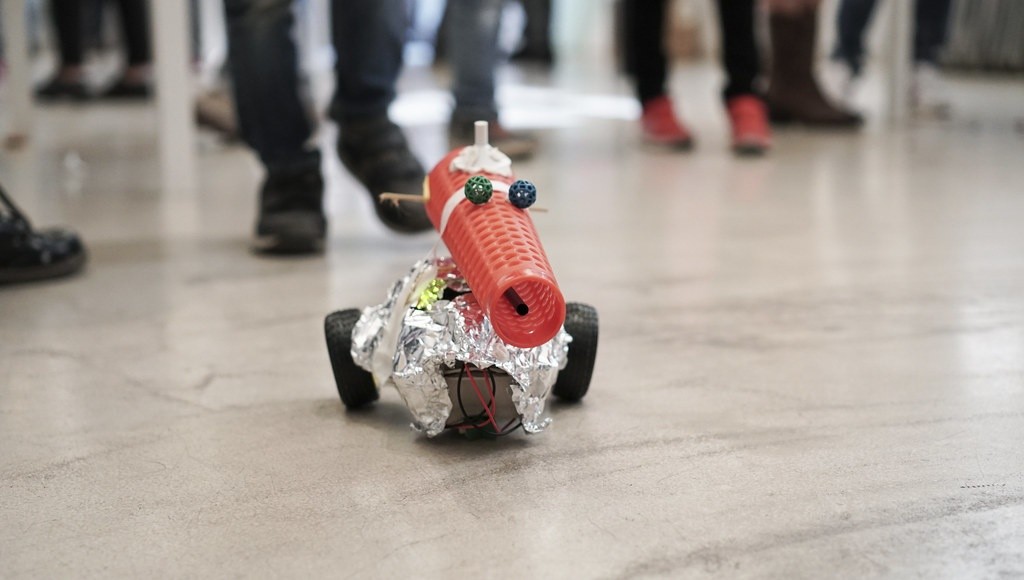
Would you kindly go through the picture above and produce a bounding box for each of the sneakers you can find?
[251,149,327,254]
[729,98,771,148]
[337,122,433,232]
[641,98,693,145]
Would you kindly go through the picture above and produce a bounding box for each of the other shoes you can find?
[98,75,152,100]
[450,121,537,156]
[34,73,95,102]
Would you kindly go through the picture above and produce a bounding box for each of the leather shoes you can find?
[0,190,88,286]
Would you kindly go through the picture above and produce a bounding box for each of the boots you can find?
[768,10,863,125]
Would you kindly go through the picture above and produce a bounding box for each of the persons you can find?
[757,0,866,132]
[26,1,159,104]
[611,0,772,155]
[827,0,958,117]
[218,1,445,261]
[195,0,544,171]
[424,1,560,88]
[0,185,88,291]
